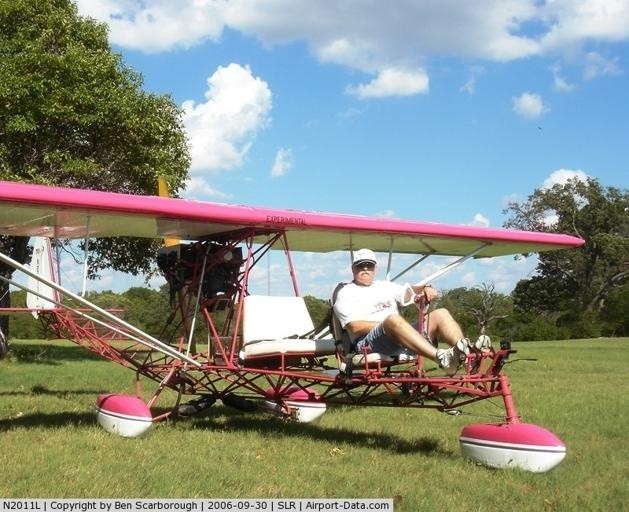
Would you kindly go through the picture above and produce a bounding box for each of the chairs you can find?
[329,280,419,366]
[242,295,338,361]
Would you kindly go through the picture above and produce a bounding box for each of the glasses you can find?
[357,262,373,268]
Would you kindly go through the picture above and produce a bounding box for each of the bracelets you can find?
[420,284,431,291]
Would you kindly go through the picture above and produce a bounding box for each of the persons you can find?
[331,246,491,377]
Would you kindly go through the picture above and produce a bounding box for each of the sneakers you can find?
[438,338,468,375]
[471,334,491,353]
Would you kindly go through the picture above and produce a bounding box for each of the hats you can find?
[352,249,378,268]
